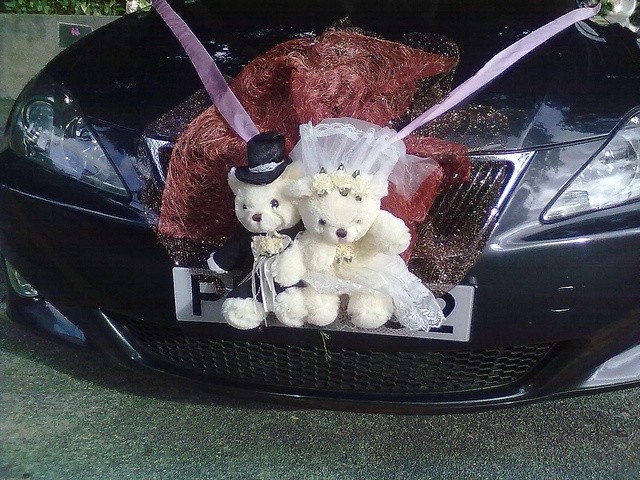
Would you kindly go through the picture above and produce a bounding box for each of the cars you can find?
[0,0,640,415]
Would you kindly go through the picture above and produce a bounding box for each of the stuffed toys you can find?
[271,118,447,332]
[206,132,306,333]
